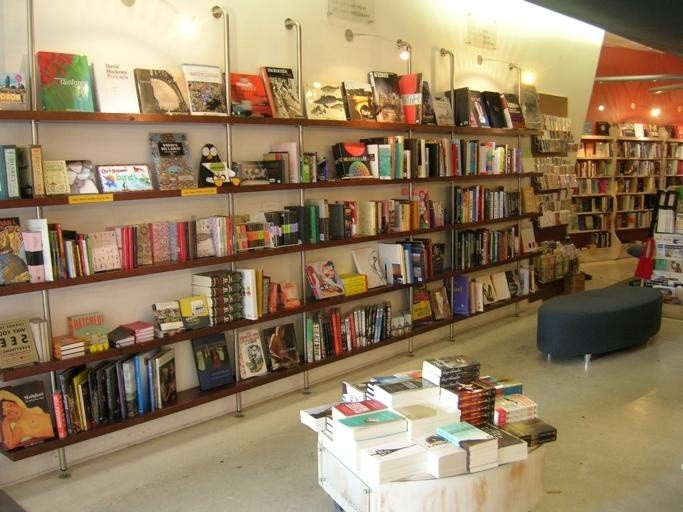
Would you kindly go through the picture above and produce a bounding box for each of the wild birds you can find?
[132,166,145,173]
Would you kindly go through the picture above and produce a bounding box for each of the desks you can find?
[317,442,544,512]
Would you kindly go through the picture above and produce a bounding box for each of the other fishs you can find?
[320,85,340,93]
[311,105,326,116]
[313,94,343,108]
[330,103,345,112]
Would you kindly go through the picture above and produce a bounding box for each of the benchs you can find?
[536,287,663,361]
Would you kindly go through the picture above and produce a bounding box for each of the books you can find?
[0,51,230,199]
[645,243,683,296]
[230,67,452,379]
[575,121,683,248]
[300,355,557,478]
[445,85,578,317]
[1,217,243,450]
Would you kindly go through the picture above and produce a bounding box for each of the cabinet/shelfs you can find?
[570,135,683,249]
[1,0,543,473]
